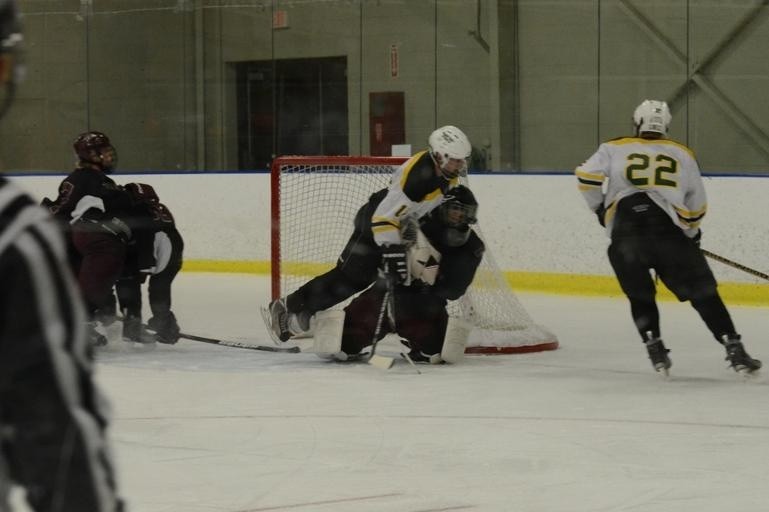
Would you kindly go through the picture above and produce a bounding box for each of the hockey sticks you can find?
[366,292,395,369]
[117,313,313,354]
[386,264,421,373]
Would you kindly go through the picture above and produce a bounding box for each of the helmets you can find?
[634,101,672,134]
[428,125,472,169]
[439,185,477,227]
[73,131,117,170]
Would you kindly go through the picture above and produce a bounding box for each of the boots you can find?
[88,322,107,346]
[646,340,671,372]
[297,311,311,331]
[269,298,291,342]
[721,334,762,370]
[122,310,179,344]
[400,348,446,364]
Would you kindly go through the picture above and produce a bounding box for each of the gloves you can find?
[692,228,701,247]
[595,195,606,228]
[380,244,407,285]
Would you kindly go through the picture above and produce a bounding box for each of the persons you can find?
[575,99,760,377]
[0,1,124,511]
[269,126,473,346]
[50,130,182,344]
[335,185,487,366]
